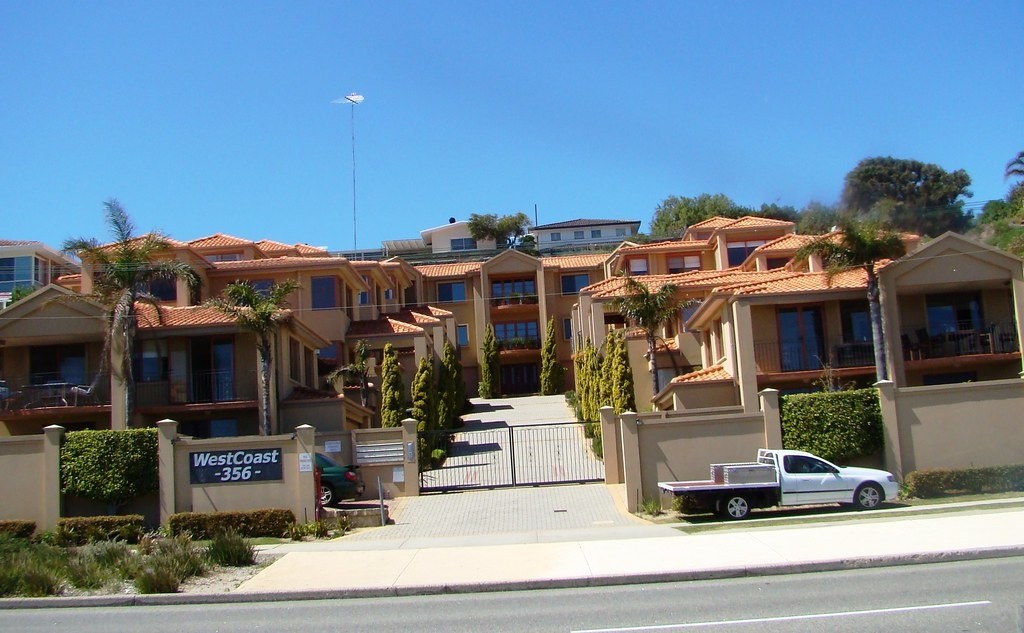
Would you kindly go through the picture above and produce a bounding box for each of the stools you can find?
[979,333,1002,354]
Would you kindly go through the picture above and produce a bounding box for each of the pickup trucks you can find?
[655,448,900,521]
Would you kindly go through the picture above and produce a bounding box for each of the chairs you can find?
[0,380,24,409]
[902,328,941,361]
[996,320,1018,351]
[69,373,103,406]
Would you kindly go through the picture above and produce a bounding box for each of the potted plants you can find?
[431,449,446,468]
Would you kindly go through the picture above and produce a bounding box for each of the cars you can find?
[313,451,362,508]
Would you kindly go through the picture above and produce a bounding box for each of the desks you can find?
[946,328,984,354]
[834,342,876,366]
[22,382,75,409]
